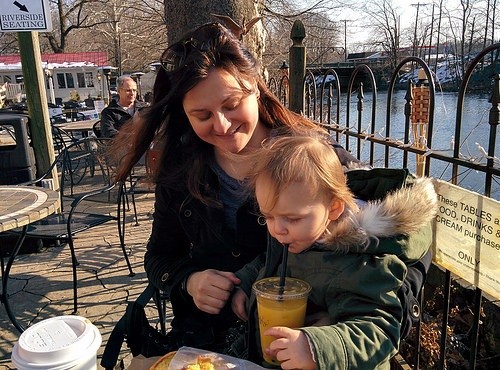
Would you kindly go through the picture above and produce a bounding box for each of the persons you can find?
[231,127,438,370]
[101,75,150,146]
[100,24,433,370]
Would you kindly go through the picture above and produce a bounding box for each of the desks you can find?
[0,185,61,333]
[60,120,101,184]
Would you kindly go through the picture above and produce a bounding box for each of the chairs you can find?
[0,112,167,370]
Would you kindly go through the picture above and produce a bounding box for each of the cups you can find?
[251,277,312,365]
[11,314,102,370]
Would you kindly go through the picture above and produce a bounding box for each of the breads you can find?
[184,358,215,370]
